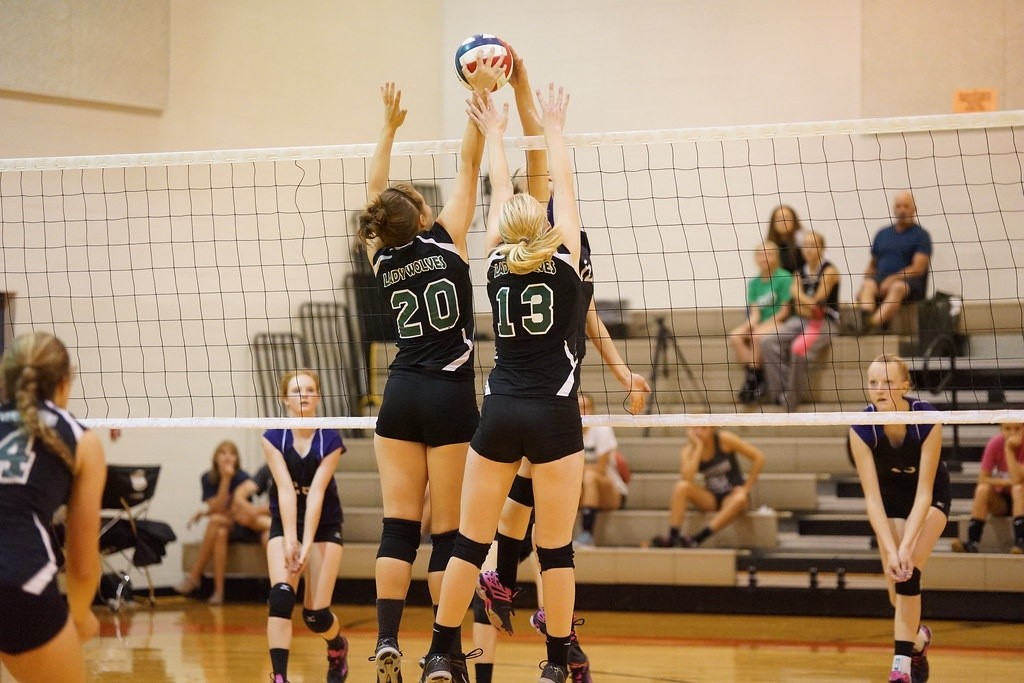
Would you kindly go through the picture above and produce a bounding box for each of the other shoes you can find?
[652,536,672,547]
[206,591,224,605]
[574,531,595,547]
[173,572,201,595]
[673,536,698,547]
[738,366,765,403]
[951,540,980,552]
[1010,538,1024,554]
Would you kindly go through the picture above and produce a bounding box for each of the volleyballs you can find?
[454,33,514,93]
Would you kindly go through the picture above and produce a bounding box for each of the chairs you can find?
[102,463,160,606]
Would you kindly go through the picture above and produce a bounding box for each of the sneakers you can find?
[530,607,589,669]
[911,624,931,683]
[374,637,403,683]
[475,570,524,638]
[327,636,348,683]
[419,654,452,683]
[539,660,569,683]
[418,652,471,683]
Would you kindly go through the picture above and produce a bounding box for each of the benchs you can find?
[180,301,1023,611]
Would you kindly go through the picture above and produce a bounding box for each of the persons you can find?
[181,441,274,606]
[731,205,840,411]
[470,529,499,683]
[651,407,764,548]
[848,191,931,333]
[849,354,952,683]
[576,392,629,545]
[952,423,1024,555]
[261,367,349,683]
[355,47,508,683]
[421,80,585,683]
[475,45,652,666]
[0,331,107,683]
